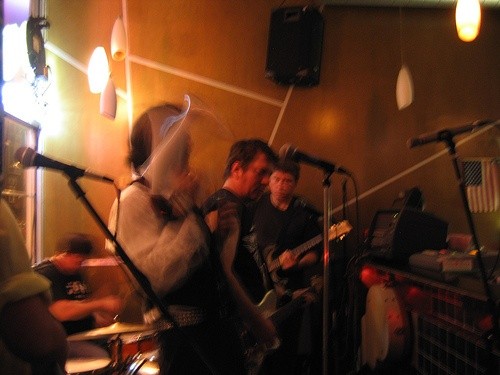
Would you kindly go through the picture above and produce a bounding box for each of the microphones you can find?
[15,147,114,183]
[278,143,347,174]
[293,200,337,224]
[409,121,491,149]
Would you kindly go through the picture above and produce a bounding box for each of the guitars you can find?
[254,275,325,349]
[356,286,404,368]
[262,219,353,274]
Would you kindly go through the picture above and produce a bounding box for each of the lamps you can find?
[395,6,414,111]
[110,11,129,63]
[455,0,482,42]
[99,81,118,121]
[87,44,111,94]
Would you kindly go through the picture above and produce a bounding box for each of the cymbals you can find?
[66,322,157,342]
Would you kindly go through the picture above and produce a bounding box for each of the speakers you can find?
[367,209,449,269]
[267,4,324,89]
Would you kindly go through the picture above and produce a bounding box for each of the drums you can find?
[107,329,159,371]
[64,356,113,375]
[127,352,160,375]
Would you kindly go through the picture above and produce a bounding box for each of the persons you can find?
[32,232,124,375]
[107,103,339,375]
[0,198,68,375]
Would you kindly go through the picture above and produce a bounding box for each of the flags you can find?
[462,157,500,213]
